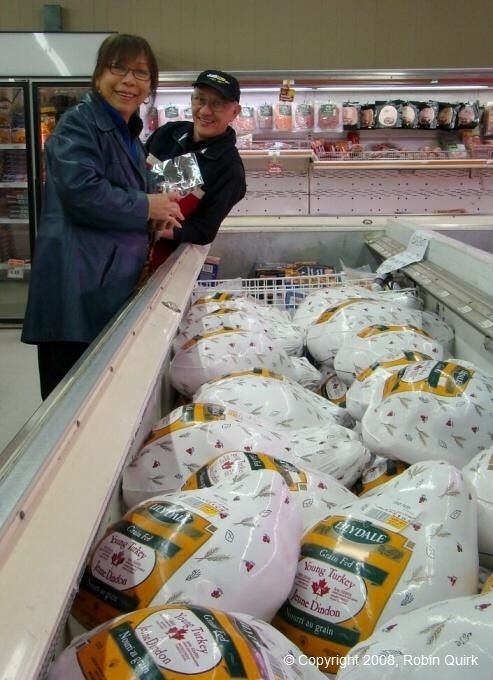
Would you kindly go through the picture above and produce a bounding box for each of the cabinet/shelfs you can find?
[135,67,493,216]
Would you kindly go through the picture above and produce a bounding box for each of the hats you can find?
[191,70,240,102]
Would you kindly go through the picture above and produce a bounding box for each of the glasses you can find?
[193,90,224,112]
[104,62,155,81]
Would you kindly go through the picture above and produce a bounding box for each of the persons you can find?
[13,34,187,403]
[145,69,246,286]
